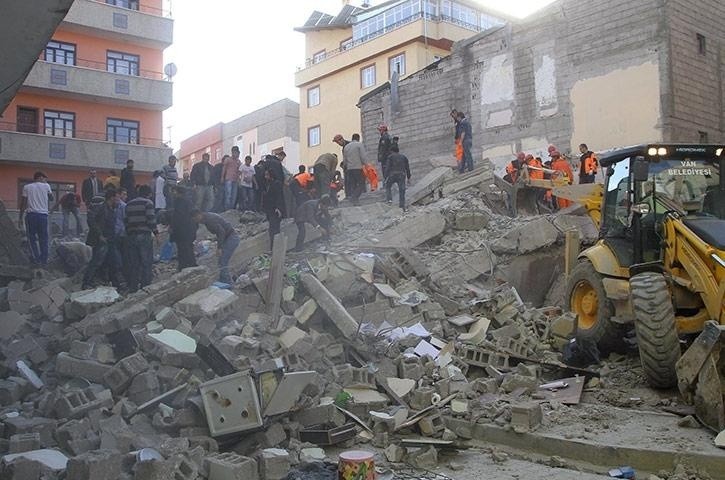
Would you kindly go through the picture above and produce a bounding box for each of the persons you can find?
[377,123,399,190]
[81,160,161,296]
[294,194,334,252]
[384,146,412,212]
[263,169,284,252]
[190,146,344,218]
[332,133,379,206]
[577,144,598,184]
[193,212,240,289]
[503,144,575,215]
[169,185,199,273]
[451,109,474,173]
[19,172,55,268]
[640,183,675,215]
[52,193,81,238]
[152,155,190,225]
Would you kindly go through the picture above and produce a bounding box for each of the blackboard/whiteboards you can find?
[564,142,724,434]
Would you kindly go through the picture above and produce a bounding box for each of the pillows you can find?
[332,134,343,142]
[378,124,387,132]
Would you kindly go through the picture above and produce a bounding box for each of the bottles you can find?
[550,151,560,157]
